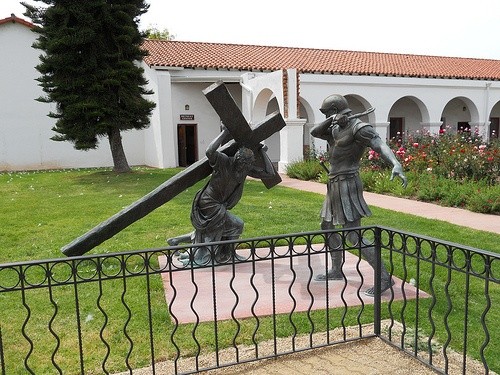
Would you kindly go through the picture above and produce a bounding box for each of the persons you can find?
[309,95,408,296]
[167,120,276,267]
